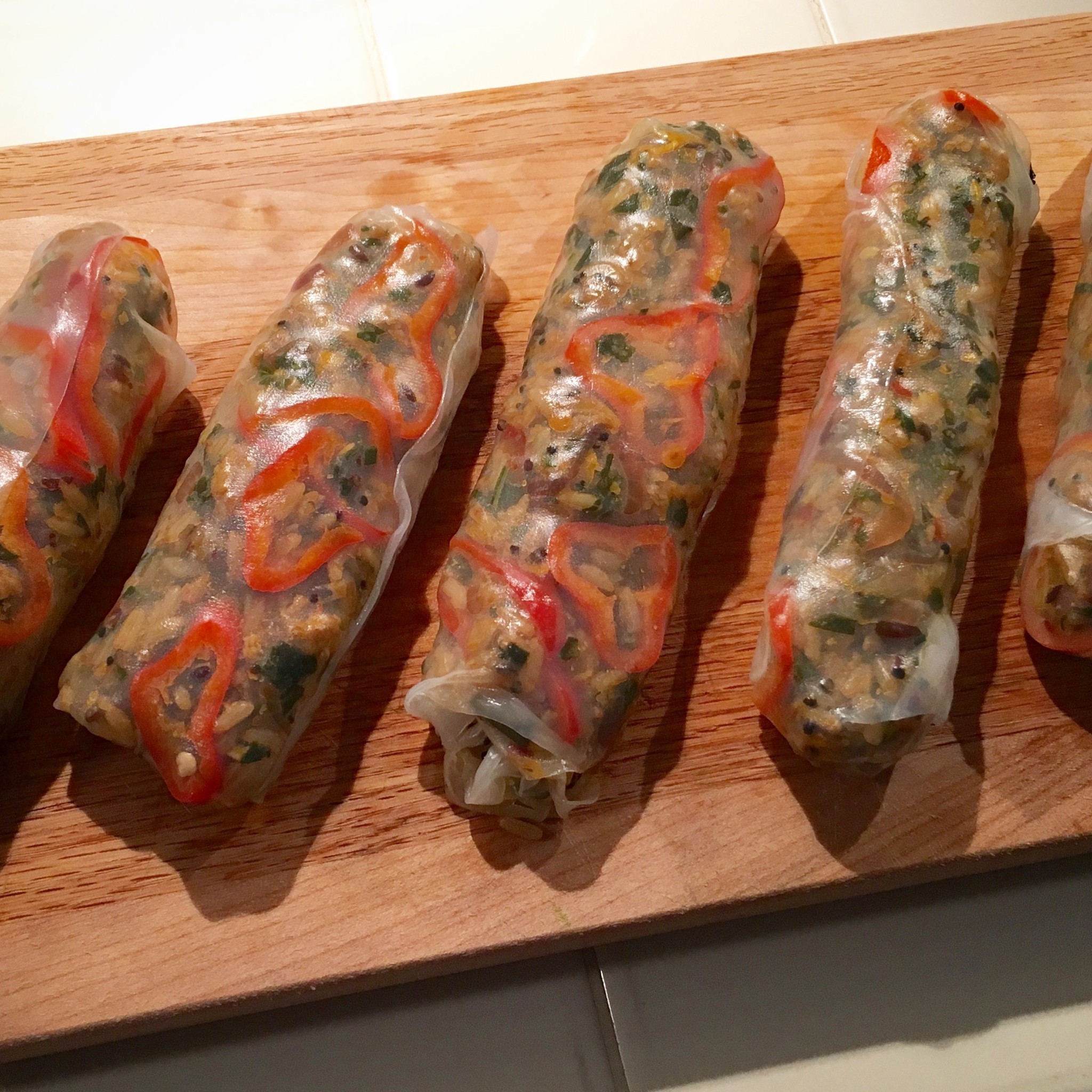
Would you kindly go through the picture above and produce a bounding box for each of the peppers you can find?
[0,86,1092,806]
